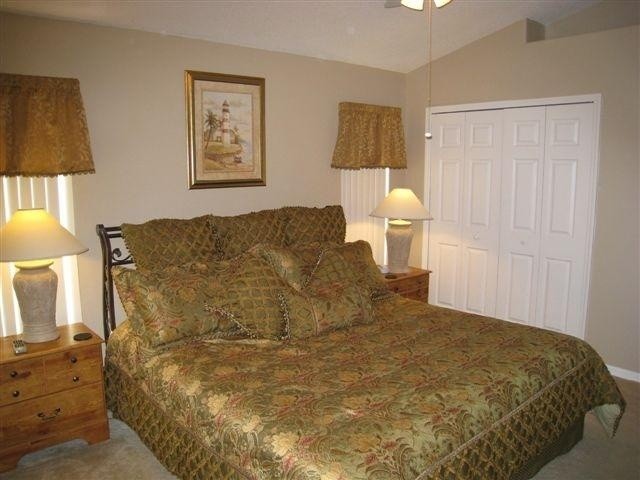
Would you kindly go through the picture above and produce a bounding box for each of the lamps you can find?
[368,188,435,273]
[0,209,89,344]
[401,0,451,138]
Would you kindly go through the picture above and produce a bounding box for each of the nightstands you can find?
[0,322,110,472]
[379,264,432,303]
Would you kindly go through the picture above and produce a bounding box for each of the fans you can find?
[384,0,401,8]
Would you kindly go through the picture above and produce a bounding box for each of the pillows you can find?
[112,261,224,356]
[211,207,288,257]
[306,240,385,299]
[286,206,349,244]
[278,278,376,339]
[205,253,304,340]
[257,239,344,291]
[119,213,222,267]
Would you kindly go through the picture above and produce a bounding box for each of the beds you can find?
[97,221,625,480]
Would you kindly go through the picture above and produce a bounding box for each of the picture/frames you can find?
[184,69,267,189]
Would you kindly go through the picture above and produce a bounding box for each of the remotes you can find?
[12,337,27,354]
